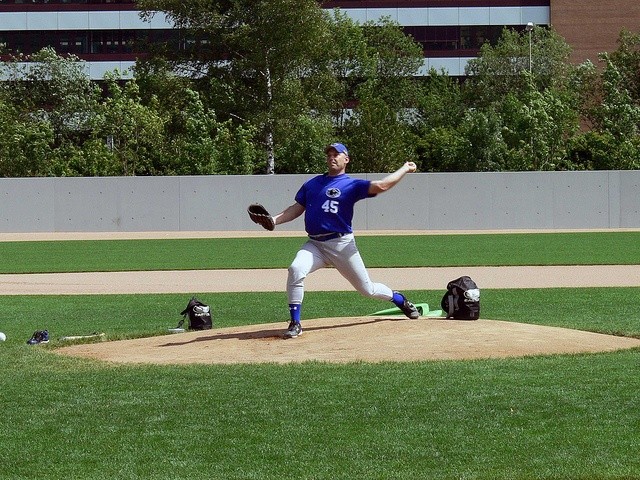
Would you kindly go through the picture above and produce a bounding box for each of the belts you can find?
[308,232,348,241]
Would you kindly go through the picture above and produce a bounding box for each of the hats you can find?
[325,142,349,156]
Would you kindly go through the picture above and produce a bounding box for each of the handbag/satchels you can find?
[175,296,212,330]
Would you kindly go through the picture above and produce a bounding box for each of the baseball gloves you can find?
[248,205,274,231]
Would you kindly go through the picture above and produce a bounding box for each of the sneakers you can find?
[282,318,303,338]
[395,291,419,319]
[26,330,50,344]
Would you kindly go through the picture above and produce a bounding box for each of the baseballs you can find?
[408,162,414,166]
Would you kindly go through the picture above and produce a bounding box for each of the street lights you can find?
[526,22,534,73]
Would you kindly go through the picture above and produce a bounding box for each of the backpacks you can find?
[441,275,480,321]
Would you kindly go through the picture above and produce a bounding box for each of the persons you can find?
[248,143,419,339]
